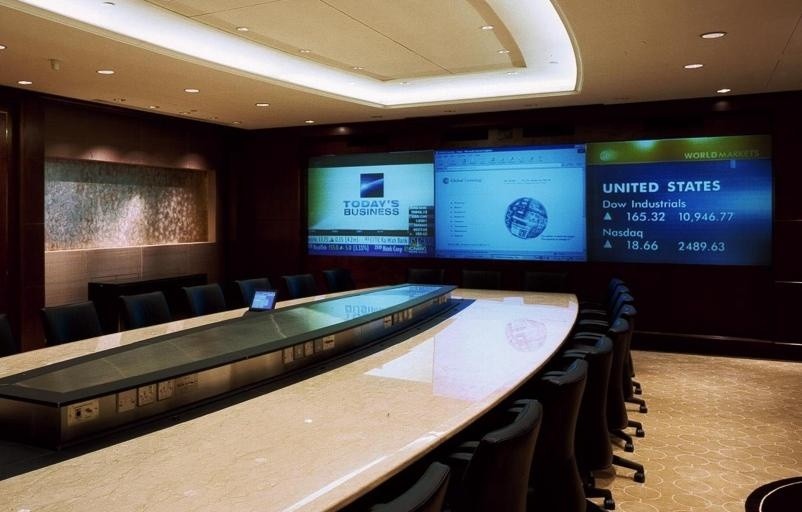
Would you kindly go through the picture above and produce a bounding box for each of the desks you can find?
[3,283,581,508]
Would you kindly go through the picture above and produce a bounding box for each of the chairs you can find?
[551,279,645,509]
[523,359,589,510]
[406,260,607,298]
[1,259,360,356]
[448,396,543,511]
[370,461,451,510]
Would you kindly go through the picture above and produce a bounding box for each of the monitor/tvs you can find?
[249,287,279,310]
